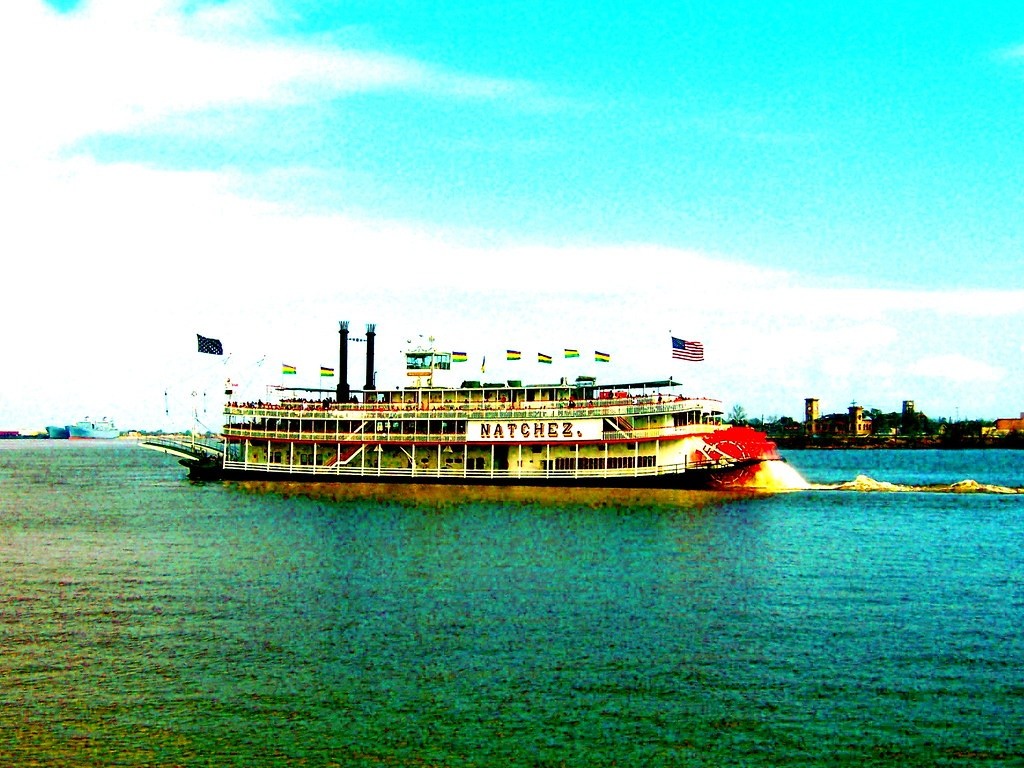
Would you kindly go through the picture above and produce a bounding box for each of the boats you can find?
[178,319,814,511]
[44,425,67,439]
[67,417,121,440]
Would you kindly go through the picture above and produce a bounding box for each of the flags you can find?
[197,333,223,355]
[594,351,610,362]
[283,364,297,374]
[564,349,580,358]
[672,336,704,361]
[452,352,467,362]
[482,356,485,373]
[538,353,552,363]
[507,350,521,360]
[321,367,335,377]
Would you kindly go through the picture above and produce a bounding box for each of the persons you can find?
[202,449,223,462]
[608,390,689,404]
[445,399,469,409]
[406,400,412,410]
[501,395,506,402]
[729,419,744,426]
[282,395,359,410]
[559,398,568,408]
[228,399,263,408]
[371,398,382,409]
